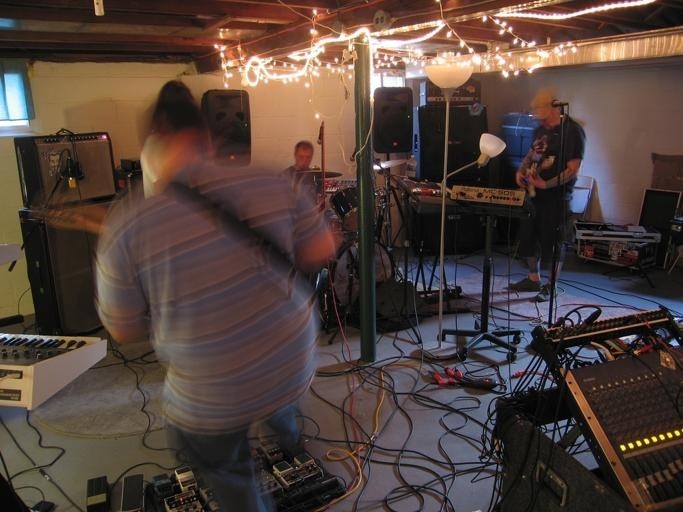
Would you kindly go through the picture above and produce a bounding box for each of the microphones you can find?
[67,159,76,188]
[551,99,568,107]
[350,150,356,161]
[317,120,324,144]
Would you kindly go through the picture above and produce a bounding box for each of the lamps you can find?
[423,62,505,360]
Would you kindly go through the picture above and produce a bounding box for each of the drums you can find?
[320,238,395,310]
[329,185,359,232]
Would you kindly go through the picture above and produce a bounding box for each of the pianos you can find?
[0,333,108,410]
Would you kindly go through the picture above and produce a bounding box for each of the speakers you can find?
[200,89,251,168]
[412,104,488,256]
[14,132,120,209]
[372,86,414,154]
[17,199,120,336]
[347,280,421,334]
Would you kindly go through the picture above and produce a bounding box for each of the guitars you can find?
[525,156,556,196]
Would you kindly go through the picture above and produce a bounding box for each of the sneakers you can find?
[536,285,557,301]
[509,278,540,291]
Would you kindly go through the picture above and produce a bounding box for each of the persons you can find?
[277,140,323,195]
[511,87,586,300]
[96,77,341,512]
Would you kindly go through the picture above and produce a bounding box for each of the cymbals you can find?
[373,160,407,171]
[297,170,343,177]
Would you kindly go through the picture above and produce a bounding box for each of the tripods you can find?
[328,247,360,347]
[376,163,423,344]
